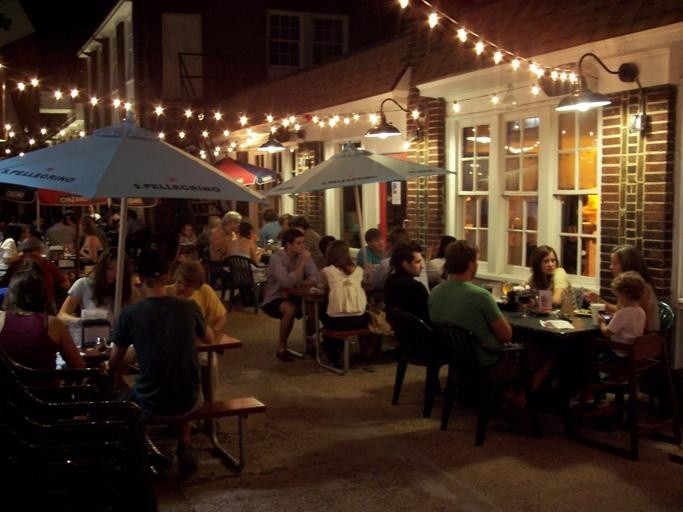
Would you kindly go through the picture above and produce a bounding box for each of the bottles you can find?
[96,337,105,353]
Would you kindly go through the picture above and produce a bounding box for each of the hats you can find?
[135,246,172,278]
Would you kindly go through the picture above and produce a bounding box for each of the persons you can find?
[0,208,269,477]
[257,209,661,437]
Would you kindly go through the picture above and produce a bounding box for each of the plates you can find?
[572,309,592,316]
[531,306,560,316]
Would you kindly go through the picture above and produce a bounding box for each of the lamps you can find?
[365,98,423,141]
[258,123,306,153]
[553,50,648,134]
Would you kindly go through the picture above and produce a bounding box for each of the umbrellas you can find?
[0,109,270,330]
[0,183,161,232]
[266,140,457,265]
[210,157,281,211]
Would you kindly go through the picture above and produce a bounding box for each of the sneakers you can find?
[598,375,627,388]
[149,451,173,472]
[176,438,199,470]
[305,342,322,356]
[275,350,294,363]
[568,395,595,409]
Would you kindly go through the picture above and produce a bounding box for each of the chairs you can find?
[383,307,451,418]
[569,334,679,463]
[0,246,268,511]
[508,289,539,310]
[654,302,676,332]
[440,321,543,447]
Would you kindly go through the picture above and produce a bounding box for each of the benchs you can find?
[258,279,311,360]
[315,322,379,373]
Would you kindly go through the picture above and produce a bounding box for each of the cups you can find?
[500,280,512,294]
[589,302,607,326]
